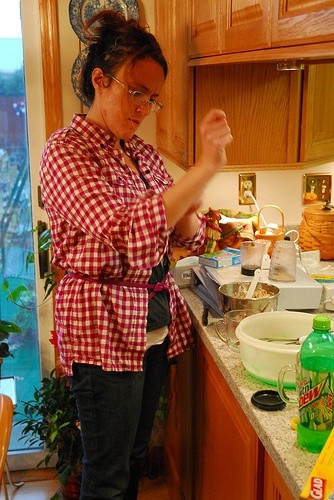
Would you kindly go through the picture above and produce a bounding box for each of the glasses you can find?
[106,73,163,112]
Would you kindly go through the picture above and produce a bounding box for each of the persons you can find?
[38,8,233,500]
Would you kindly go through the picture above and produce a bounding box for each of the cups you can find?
[239,242,264,275]
[277,351,301,406]
[215,310,257,351]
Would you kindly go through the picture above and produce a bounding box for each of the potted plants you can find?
[13,366,84,500]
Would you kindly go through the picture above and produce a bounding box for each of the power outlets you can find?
[302,173,332,207]
[239,173,257,205]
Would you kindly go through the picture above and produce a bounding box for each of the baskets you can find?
[256,204,285,258]
[297,203,334,261]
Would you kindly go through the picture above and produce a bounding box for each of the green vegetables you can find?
[217,209,257,219]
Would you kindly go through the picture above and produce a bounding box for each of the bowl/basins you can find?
[235,311,316,389]
[218,282,280,315]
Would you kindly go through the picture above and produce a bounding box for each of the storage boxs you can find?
[199,247,241,268]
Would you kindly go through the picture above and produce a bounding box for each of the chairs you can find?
[0,395,24,500]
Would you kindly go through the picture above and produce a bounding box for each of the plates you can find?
[70,46,90,108]
[68,0,139,46]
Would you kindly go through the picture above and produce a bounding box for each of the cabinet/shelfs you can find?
[164,334,298,500]
[152,0,334,173]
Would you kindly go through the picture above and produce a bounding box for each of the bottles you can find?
[268,239,296,283]
[296,316,334,452]
